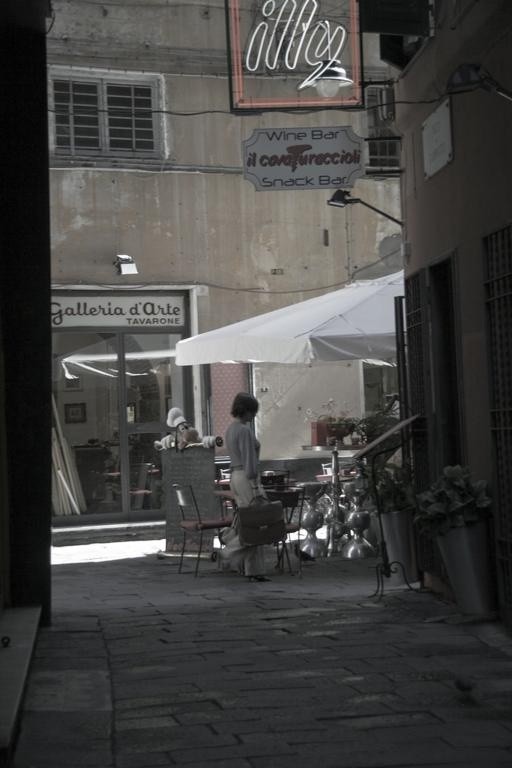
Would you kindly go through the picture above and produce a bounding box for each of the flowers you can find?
[301,398,355,423]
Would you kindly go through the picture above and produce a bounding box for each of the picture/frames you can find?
[65,403,85,424]
[63,375,82,392]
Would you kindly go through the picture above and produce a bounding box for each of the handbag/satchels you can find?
[236,496,287,547]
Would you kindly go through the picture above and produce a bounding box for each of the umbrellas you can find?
[175,268,408,368]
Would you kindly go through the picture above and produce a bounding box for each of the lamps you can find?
[447,64,512,103]
[327,189,403,226]
[112,255,139,275]
[299,59,353,96]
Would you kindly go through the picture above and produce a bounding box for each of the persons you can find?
[217,390,277,582]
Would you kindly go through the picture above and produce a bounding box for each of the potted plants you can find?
[414,463,490,613]
[345,462,420,587]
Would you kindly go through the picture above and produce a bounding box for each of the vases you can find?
[311,422,351,445]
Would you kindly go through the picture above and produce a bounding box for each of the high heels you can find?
[248,574,273,582]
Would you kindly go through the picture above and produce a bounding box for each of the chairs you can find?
[173,462,349,577]
